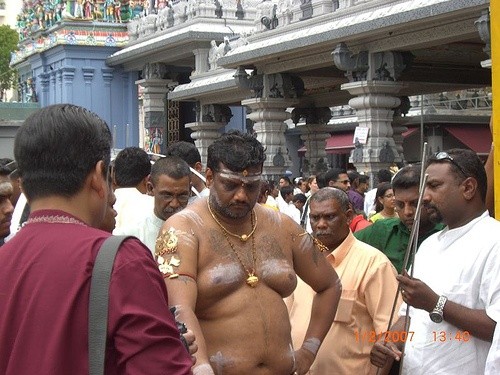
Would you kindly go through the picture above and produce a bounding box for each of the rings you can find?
[402,290,404,294]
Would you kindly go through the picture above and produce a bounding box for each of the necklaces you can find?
[211,209,259,287]
[206,196,258,241]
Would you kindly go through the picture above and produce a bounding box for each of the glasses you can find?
[335,180,350,184]
[160,194,191,202]
[384,194,392,198]
[432,152,467,177]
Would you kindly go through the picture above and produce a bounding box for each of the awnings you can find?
[444,126,493,161]
[297,127,420,156]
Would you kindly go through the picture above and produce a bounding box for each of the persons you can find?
[0,141,447,278]
[74,0,168,24]
[281,187,404,375]
[155,129,343,375]
[16,0,67,40]
[0,104,198,375]
[368,148,500,375]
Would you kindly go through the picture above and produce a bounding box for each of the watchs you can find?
[430,294,448,323]
